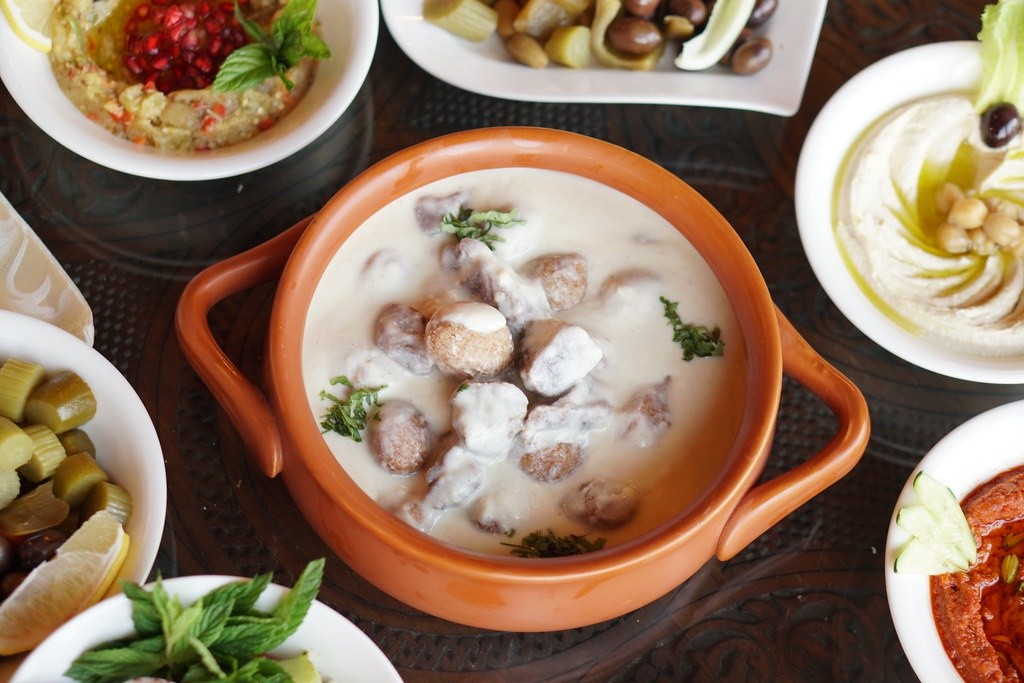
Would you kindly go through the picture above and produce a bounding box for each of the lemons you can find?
[0,508,131,656]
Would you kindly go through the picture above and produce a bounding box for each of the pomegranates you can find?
[118,0,249,91]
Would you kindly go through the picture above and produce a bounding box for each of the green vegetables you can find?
[317,211,725,561]
[63,557,328,683]
[213,0,334,95]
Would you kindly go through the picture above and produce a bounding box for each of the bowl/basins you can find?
[0,311,169,683]
[0,0,380,182]
[175,125,872,633]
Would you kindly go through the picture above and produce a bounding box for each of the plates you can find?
[6,572,405,683]
[793,40,1024,383]
[381,0,829,117]
[885,398,1024,683]
